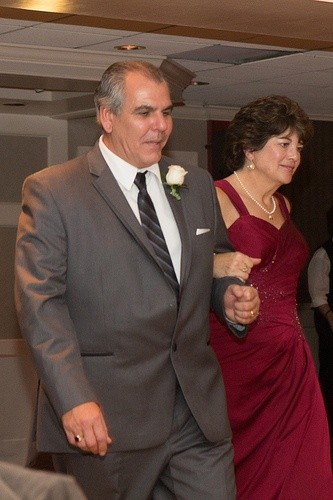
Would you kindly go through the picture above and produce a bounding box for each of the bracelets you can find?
[325,309,332,318]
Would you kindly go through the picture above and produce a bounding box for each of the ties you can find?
[133,170,182,298]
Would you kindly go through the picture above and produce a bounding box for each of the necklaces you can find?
[233,171,277,220]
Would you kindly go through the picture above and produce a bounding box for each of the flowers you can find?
[160,164,190,200]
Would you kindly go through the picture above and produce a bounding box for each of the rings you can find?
[250,310,256,317]
[75,435,85,443]
[242,266,249,272]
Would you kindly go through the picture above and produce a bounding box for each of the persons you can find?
[307,206,333,446]
[213,94,333,500]
[15,59,260,500]
